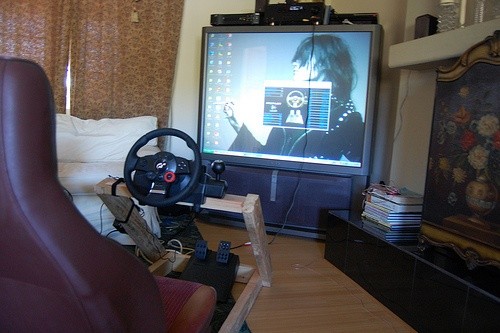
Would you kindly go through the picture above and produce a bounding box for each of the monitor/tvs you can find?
[196,25,381,177]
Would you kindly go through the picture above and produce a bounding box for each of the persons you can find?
[223,34,365,163]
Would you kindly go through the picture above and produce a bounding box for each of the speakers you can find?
[414,14,438,39]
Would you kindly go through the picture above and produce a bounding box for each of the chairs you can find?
[0,57,217,333]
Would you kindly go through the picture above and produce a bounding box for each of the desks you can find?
[325,209,500,332]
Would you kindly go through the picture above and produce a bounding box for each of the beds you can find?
[56,113,160,246]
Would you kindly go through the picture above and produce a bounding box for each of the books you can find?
[361,185,424,241]
